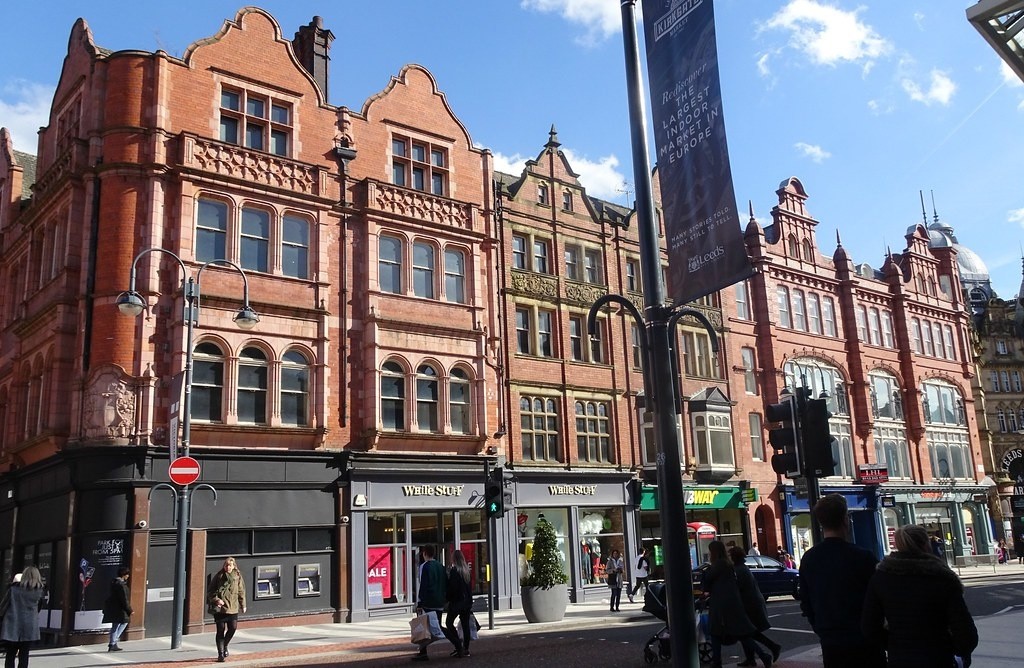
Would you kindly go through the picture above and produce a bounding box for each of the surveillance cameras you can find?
[343,516,349,522]
[140,520,147,527]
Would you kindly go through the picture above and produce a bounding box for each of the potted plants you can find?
[520,518,569,622]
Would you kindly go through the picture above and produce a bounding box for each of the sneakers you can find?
[411,653,429,662]
[450,649,471,658]
[628,593,634,603]
[224,647,229,657]
[218,654,225,662]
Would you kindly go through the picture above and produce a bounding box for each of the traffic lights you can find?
[767,396,801,479]
[486,467,503,517]
[503,472,514,515]
[803,399,839,478]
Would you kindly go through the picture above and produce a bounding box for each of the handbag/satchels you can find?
[470,610,481,632]
[455,613,480,642]
[207,602,221,615]
[419,608,446,646]
[410,612,432,644]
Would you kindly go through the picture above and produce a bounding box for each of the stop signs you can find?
[169,456,200,485]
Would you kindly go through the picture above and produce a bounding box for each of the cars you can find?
[692,555,801,603]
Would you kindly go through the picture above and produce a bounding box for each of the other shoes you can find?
[616,608,620,612]
[762,653,772,668]
[773,645,781,663]
[737,659,757,666]
[108,643,123,652]
[610,609,615,612]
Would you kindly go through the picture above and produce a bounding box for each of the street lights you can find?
[116,247,261,651]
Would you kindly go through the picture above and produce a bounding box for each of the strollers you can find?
[642,581,714,666]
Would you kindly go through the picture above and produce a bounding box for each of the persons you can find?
[777,545,796,569]
[1015,532,1024,564]
[628,548,651,602]
[796,494,887,668]
[867,524,979,668]
[207,557,247,661]
[997,537,1010,564]
[699,540,782,668]
[606,550,624,612]
[0,566,44,668]
[411,544,473,660]
[518,530,534,565]
[102,567,135,652]
[583,533,606,584]
